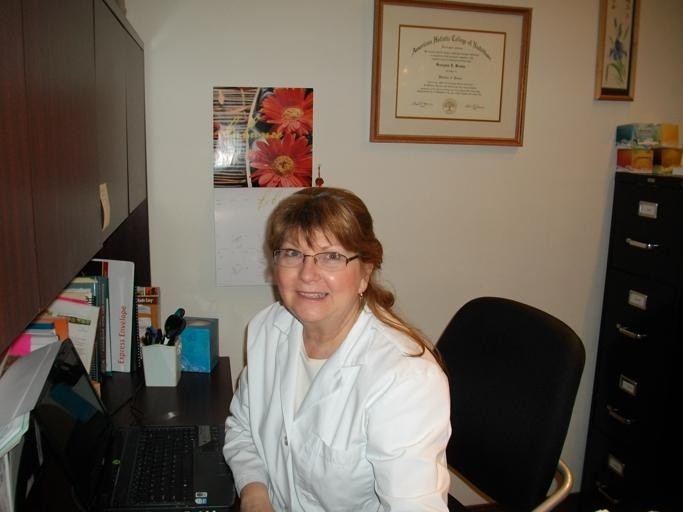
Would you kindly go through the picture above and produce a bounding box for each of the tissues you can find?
[175,316,219,372]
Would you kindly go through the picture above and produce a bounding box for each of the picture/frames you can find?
[594,0,642,102]
[369,0,533,146]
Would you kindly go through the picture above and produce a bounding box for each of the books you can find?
[1,258,160,382]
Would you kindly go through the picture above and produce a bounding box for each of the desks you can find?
[103,356,241,511]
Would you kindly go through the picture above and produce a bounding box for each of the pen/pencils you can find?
[140,308,185,346]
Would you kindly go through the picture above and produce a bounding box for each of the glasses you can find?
[271,247,360,272]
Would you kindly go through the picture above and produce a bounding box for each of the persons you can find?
[222,187,452,511]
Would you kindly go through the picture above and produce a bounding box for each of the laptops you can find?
[30,337,235,512]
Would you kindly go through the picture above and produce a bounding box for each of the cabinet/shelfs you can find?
[580,170,683,512]
[0,0,104,360]
[92,0,148,243]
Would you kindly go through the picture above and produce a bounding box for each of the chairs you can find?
[432,295,586,512]
